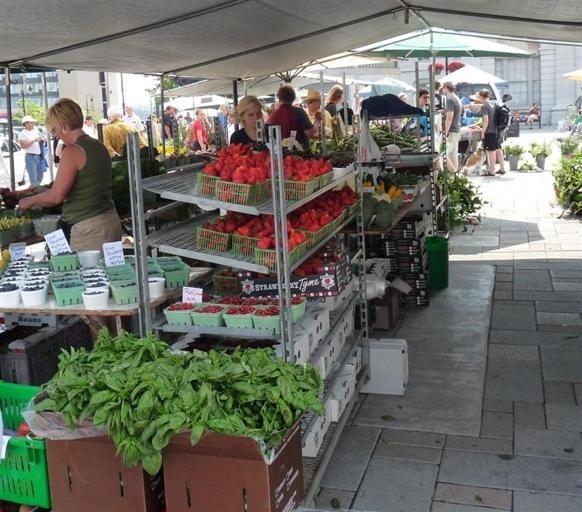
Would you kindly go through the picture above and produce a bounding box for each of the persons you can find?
[230,95,267,146]
[462,89,505,176]
[55,139,64,157]
[399,80,462,175]
[18,98,125,346]
[164,106,212,150]
[82,116,96,138]
[19,116,47,187]
[324,86,353,125]
[300,91,332,127]
[103,106,147,158]
[526,103,539,128]
[266,85,316,146]
[218,106,235,142]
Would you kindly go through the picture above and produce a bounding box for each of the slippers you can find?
[482,170,505,176]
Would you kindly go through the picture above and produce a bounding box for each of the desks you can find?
[1,201,170,250]
[0,267,211,348]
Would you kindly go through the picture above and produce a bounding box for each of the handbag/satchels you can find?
[493,102,510,130]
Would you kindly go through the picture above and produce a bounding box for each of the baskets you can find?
[0,379,52,509]
[297,197,363,247]
[197,169,333,205]
[211,273,236,293]
[198,227,308,271]
[374,211,431,307]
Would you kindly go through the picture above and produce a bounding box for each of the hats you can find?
[469,89,489,101]
[442,81,454,88]
[301,90,321,102]
[22,116,36,123]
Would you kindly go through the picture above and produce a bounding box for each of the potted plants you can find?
[528,143,551,170]
[505,143,523,170]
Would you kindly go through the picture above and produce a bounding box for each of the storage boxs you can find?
[45,438,165,512]
[162,416,305,511]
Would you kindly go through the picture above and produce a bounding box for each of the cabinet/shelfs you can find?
[126,115,370,511]
[362,100,450,243]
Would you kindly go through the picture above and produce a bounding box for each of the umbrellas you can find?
[561,61,581,85]
[334,73,418,94]
[355,27,535,62]
[424,63,509,95]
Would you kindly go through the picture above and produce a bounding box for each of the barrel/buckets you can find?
[424,236,449,290]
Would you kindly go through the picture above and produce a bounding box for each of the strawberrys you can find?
[197,142,360,269]
